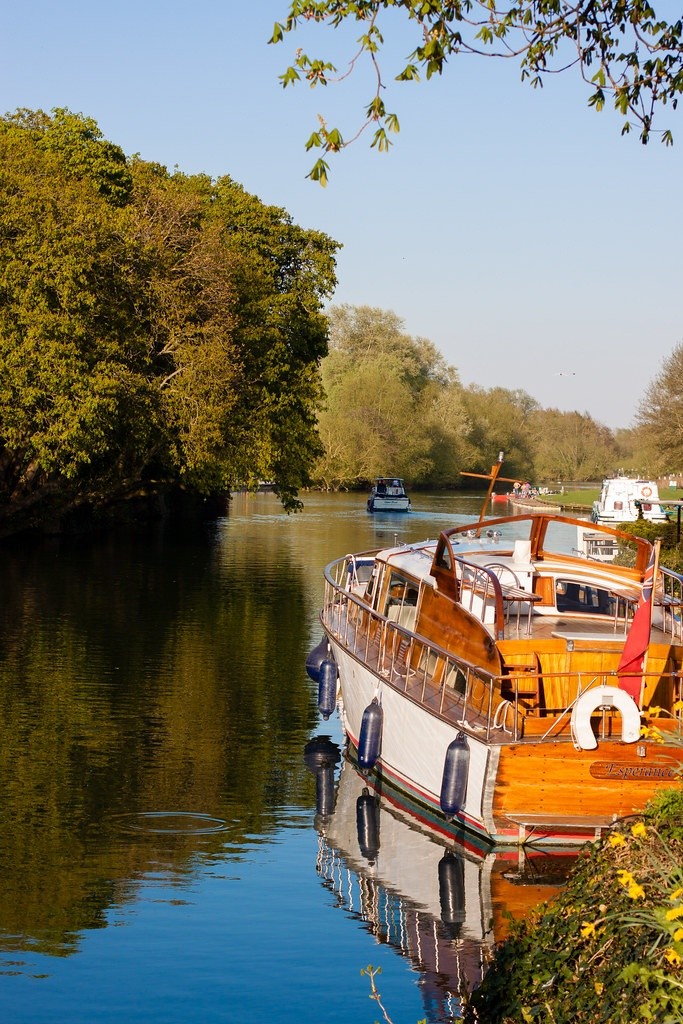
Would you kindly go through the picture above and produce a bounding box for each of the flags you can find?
[617,548,655,710]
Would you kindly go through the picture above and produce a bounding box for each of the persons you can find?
[513,481,538,500]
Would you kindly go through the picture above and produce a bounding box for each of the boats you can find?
[305,514,682,845]
[591,475,666,531]
[576,517,618,564]
[303,729,640,1024]
[367,478,412,513]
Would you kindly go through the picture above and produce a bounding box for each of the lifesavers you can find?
[569,683,642,751]
